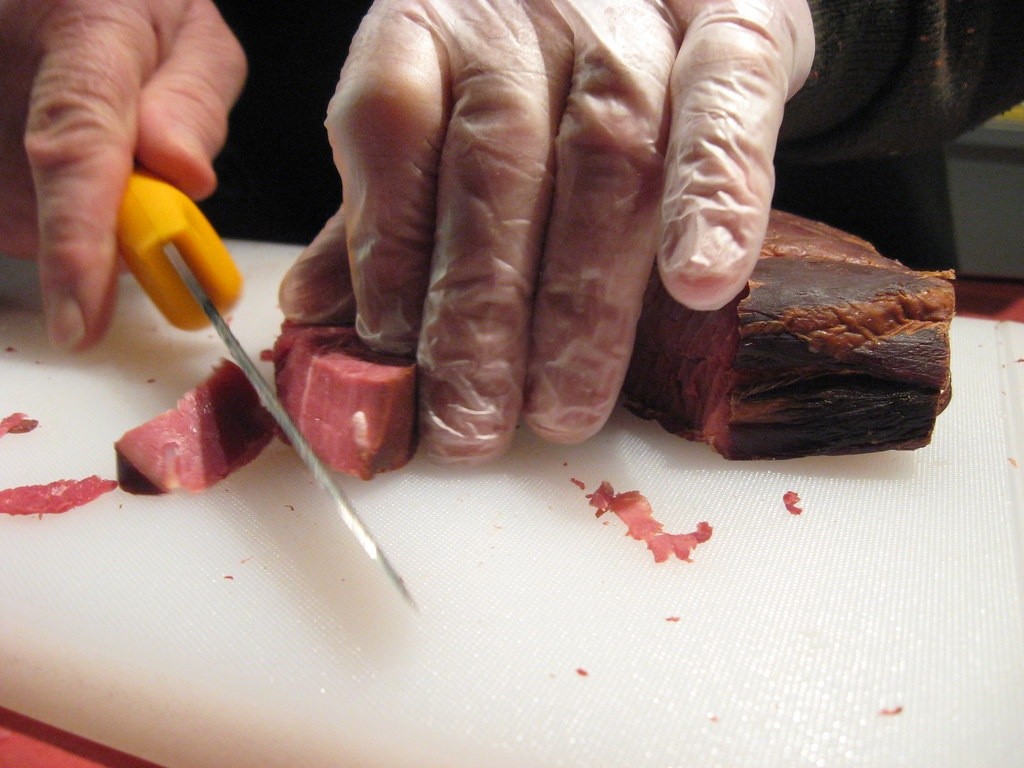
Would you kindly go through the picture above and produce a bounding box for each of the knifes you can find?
[117,170,418,614]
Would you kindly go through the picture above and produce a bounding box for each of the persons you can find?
[0,0,1024,469]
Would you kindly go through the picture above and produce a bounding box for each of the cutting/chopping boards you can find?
[0,239,1024,768]
